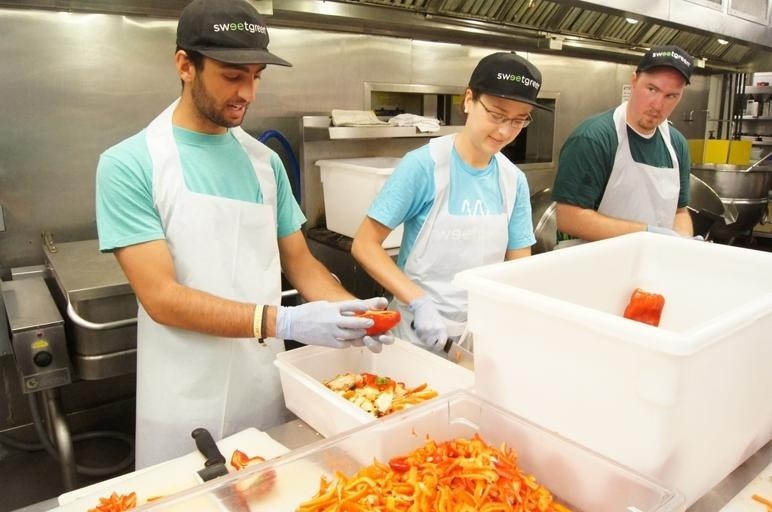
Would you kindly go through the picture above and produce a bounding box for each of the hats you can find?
[636,44,694,87]
[176,0,294,68]
[469,52,553,112]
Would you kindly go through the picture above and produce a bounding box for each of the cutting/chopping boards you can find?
[58,424,337,512]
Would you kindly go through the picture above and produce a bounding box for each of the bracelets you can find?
[253,303,268,343]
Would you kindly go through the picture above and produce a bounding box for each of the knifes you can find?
[409,317,474,364]
[190,425,250,511]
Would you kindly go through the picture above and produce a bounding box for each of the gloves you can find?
[275,299,375,349]
[646,224,705,241]
[408,294,449,354]
[350,296,398,354]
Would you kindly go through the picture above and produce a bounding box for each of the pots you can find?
[689,160,771,241]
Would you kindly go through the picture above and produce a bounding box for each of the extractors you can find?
[3,1,771,75]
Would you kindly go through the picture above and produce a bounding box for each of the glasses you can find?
[474,95,533,129]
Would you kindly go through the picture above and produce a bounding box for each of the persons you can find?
[349,49,545,366]
[93,2,399,470]
[549,43,697,240]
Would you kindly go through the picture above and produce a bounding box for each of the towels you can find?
[388,110,441,133]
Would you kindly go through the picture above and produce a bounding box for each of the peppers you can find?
[624,287,664,327]
[356,309,400,337]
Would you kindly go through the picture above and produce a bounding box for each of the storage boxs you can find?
[453,228,772,511]
[273,330,474,467]
[123,391,685,511]
[313,154,407,251]
